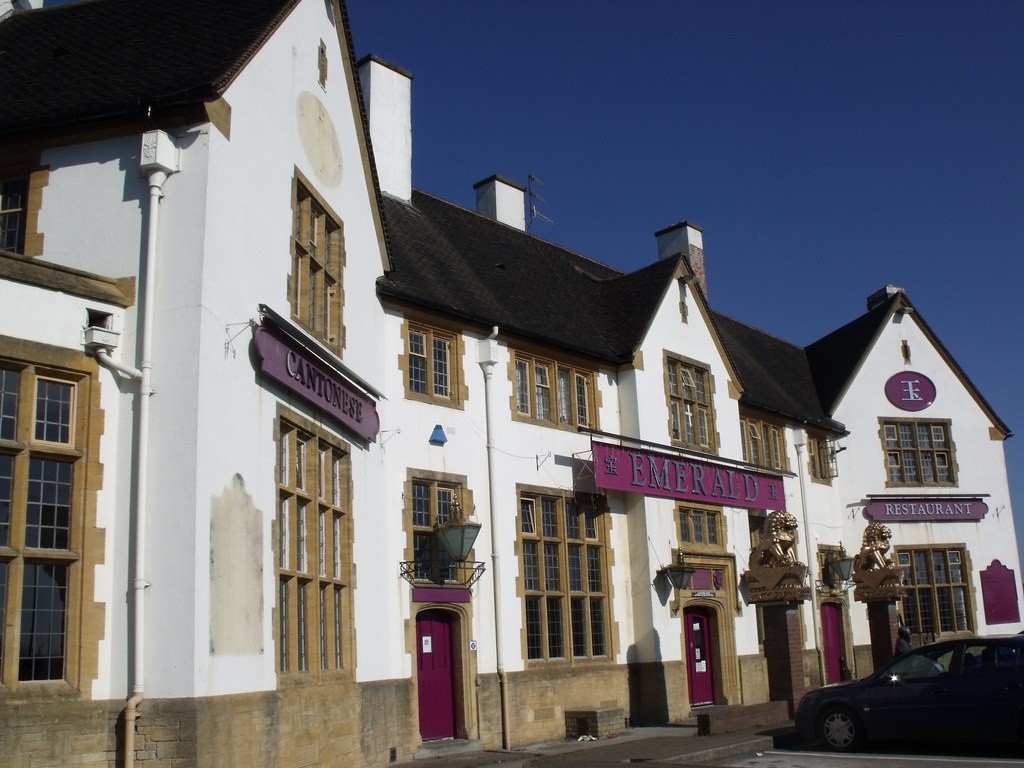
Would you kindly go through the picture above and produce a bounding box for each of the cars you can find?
[795,633,1024,758]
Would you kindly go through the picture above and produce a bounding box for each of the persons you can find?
[894,627,915,657]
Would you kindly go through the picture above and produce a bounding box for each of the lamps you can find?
[656,546,697,591]
[828,539,856,583]
[435,493,482,563]
